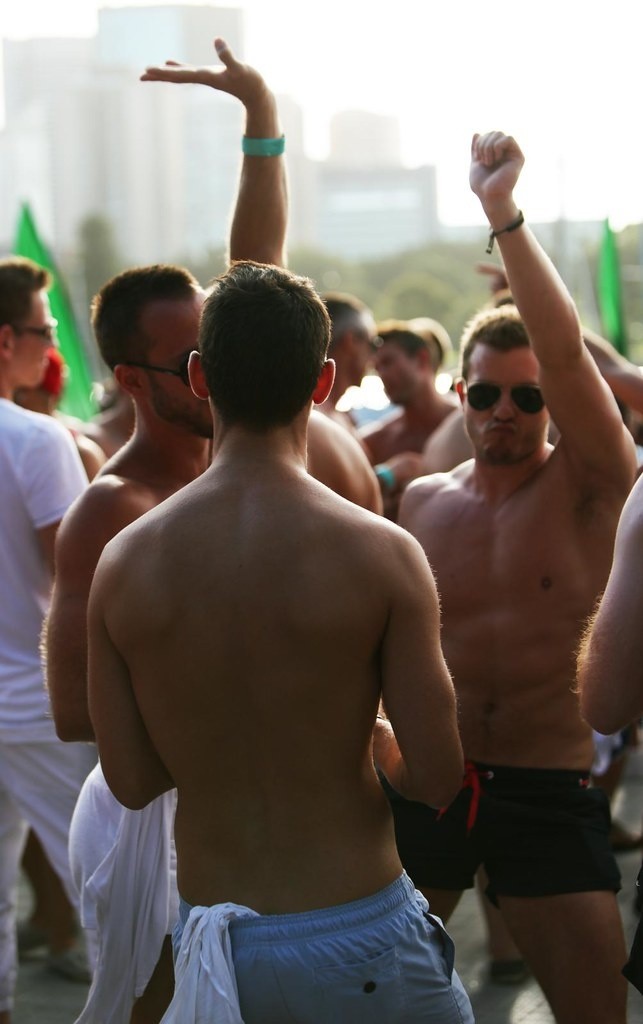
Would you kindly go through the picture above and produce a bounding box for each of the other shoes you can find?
[591,791,643,851]
[48,948,95,983]
[489,955,528,984]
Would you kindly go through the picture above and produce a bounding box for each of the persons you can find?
[88,262,474,1023]
[44,39,290,1024]
[1,258,135,1024]
[426,264,642,993]
[306,294,461,887]
[396,130,639,1023]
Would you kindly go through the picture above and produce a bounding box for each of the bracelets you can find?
[377,467,394,487]
[242,136,285,156]
[486,210,524,253]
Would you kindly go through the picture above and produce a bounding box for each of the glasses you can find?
[129,354,190,389]
[461,377,546,414]
[14,318,56,346]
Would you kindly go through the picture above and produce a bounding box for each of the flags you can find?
[14,203,98,421]
[596,218,626,344]
